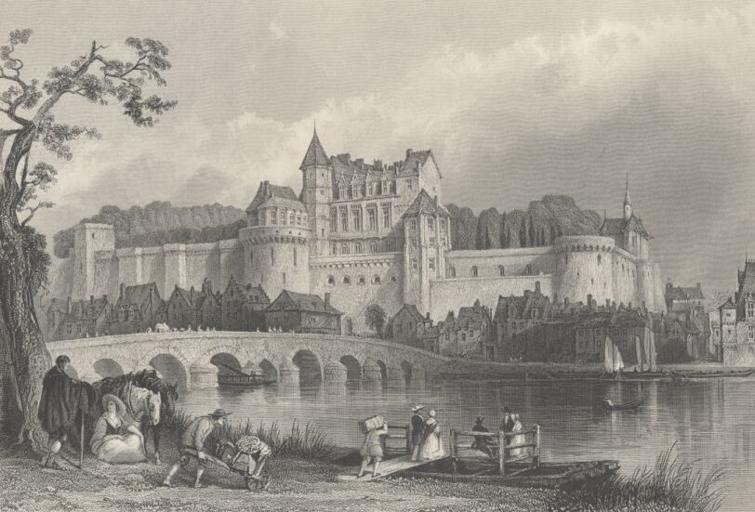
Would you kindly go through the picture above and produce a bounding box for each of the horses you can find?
[88,365,179,466]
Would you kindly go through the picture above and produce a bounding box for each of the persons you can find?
[419,409,445,459]
[510,411,529,461]
[357,415,387,478]
[471,415,491,454]
[161,408,228,487]
[38,353,96,471]
[87,393,152,464]
[499,405,512,457]
[411,403,422,461]
